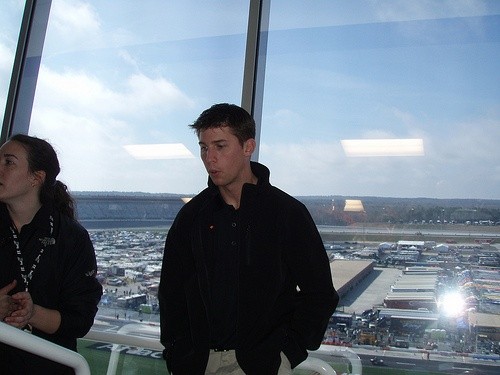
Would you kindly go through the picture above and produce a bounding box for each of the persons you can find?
[104,288,161,320]
[0,133,103,375]
[155,103,341,375]
[328,326,500,359]
